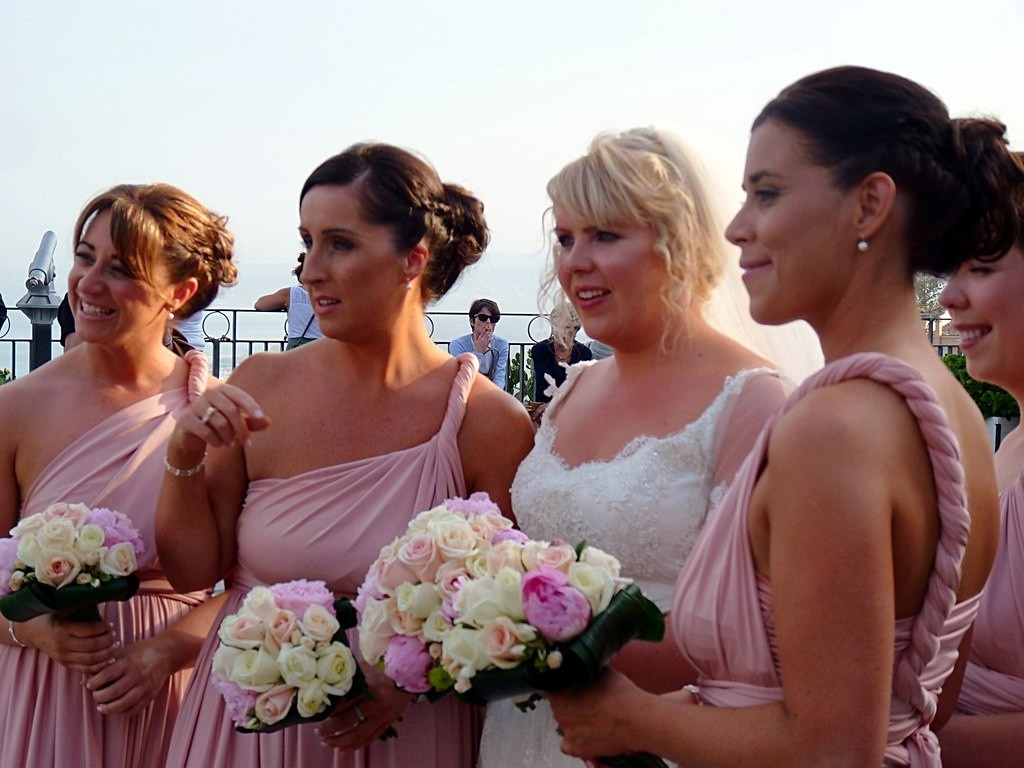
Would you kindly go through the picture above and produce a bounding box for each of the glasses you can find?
[474,313,498,323]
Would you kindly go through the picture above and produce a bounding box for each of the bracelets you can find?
[164,452,208,476]
[9,621,25,647]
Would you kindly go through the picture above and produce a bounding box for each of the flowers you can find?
[428,522,672,768]
[355,491,531,705]
[209,579,405,742]
[0,500,146,692]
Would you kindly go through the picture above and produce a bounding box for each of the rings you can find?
[203,407,214,423]
[354,706,364,721]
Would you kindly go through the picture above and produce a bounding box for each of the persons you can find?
[0,182,231,768]
[174,310,205,350]
[155,144,535,768]
[726,68,1024,768]
[531,303,593,420]
[934,152,1024,768]
[253,252,325,350]
[449,299,509,389]
[481,125,797,768]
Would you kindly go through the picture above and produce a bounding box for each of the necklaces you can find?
[556,351,571,360]
